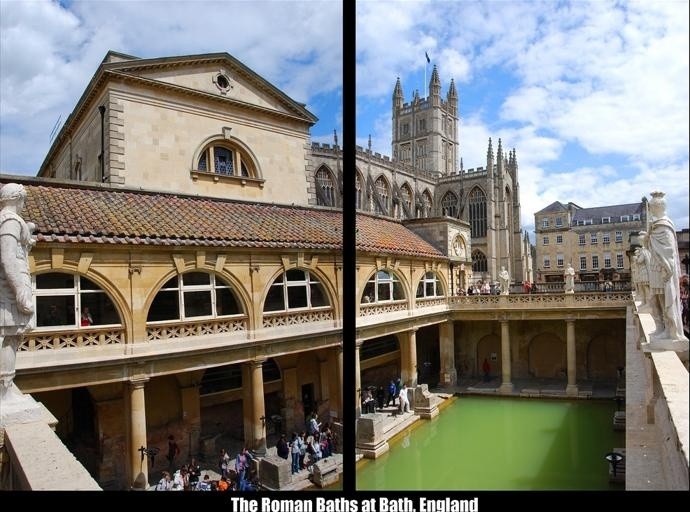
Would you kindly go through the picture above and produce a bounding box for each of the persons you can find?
[0,183,36,404]
[483,358,491,382]
[499,266,509,293]
[631,234,652,309]
[81,306,93,326]
[363,292,372,303]
[155,376,410,492]
[564,264,575,291]
[457,281,500,296]
[604,278,613,291]
[643,193,684,338]
[525,283,536,294]
